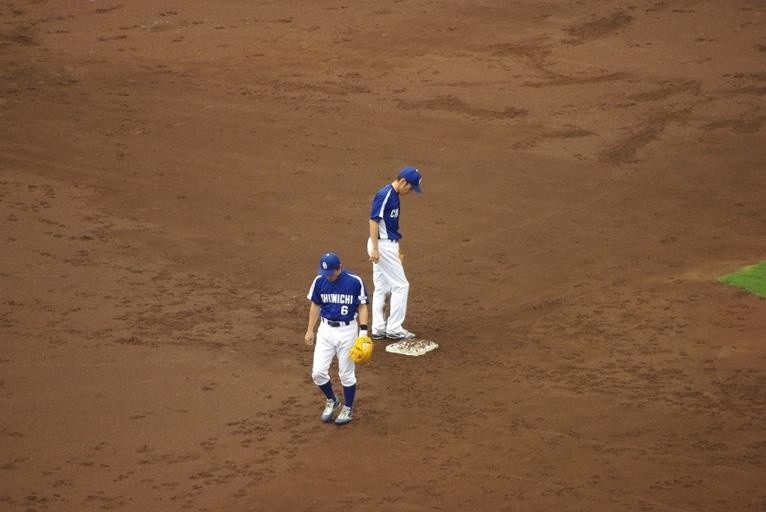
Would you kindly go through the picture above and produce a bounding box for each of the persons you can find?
[304,252,369,424]
[366,167,423,340]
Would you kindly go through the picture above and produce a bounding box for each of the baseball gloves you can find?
[349,336,374,365]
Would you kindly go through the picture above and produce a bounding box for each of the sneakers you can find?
[372,331,385,339]
[336,405,353,423]
[321,395,341,421]
[386,331,415,339]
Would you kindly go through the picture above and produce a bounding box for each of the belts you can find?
[322,318,349,327]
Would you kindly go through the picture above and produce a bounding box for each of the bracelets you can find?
[359,325,368,330]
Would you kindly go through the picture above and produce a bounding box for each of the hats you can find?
[317,252,340,276]
[401,168,421,192]
